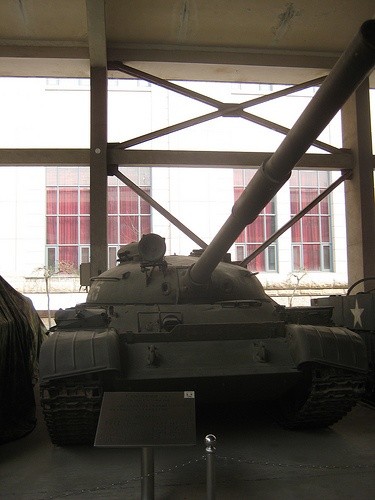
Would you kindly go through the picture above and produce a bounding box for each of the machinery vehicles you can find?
[1,18,375,450]
